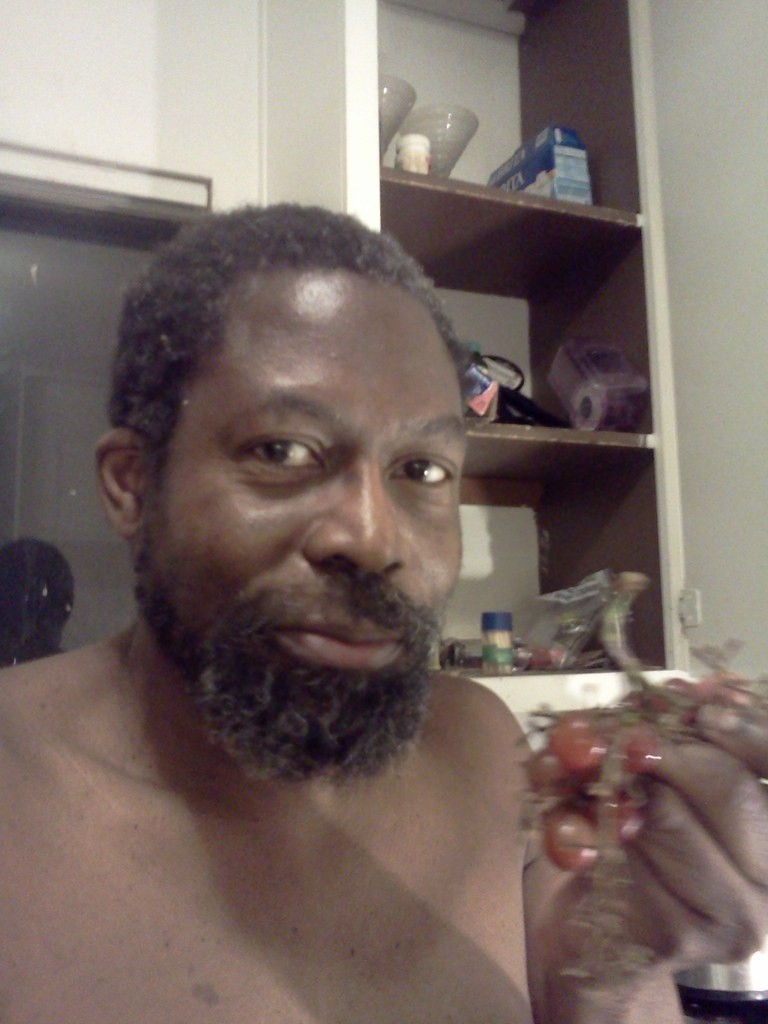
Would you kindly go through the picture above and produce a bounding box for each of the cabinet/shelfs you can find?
[263,1,694,712]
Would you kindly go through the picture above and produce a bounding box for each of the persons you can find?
[1,205,768,1024]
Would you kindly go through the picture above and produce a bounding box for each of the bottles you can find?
[481,612,515,677]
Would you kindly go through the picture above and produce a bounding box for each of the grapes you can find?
[525,677,763,878]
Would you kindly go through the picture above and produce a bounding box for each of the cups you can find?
[393,133,430,174]
[378,74,417,165]
[399,104,478,177]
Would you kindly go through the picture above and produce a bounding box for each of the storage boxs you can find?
[487,126,594,203]
